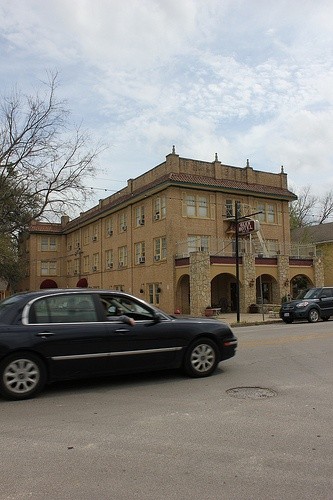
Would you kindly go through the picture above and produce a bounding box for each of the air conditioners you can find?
[122,226,127,231]
[92,265,96,270]
[118,261,123,266]
[154,253,160,261]
[108,262,113,268]
[154,214,159,220]
[139,220,144,225]
[139,256,144,263]
[91,235,97,241]
[108,230,112,235]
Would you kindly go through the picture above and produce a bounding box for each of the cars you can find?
[0,286,240,402]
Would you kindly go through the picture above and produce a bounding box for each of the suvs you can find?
[278,286,333,323]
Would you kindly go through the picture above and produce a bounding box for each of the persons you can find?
[96,301,136,327]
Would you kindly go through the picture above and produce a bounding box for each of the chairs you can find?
[79,301,95,323]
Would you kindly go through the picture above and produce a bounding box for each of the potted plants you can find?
[205,305,213,317]
